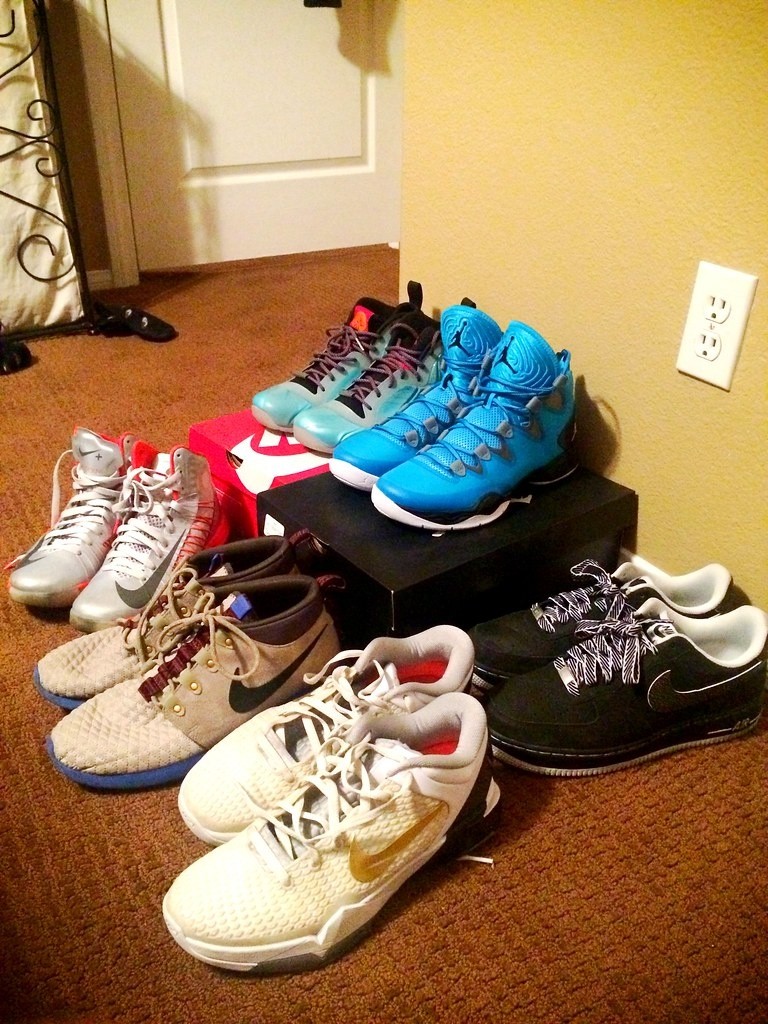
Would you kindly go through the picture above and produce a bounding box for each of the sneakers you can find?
[370,321,580,530]
[293,296,477,455]
[34,528,311,710]
[466,557,734,692]
[47,574,348,790]
[162,692,501,974]
[178,625,474,847]
[4,426,135,608]
[70,440,231,633]
[328,297,502,491]
[251,280,424,432]
[481,595,768,777]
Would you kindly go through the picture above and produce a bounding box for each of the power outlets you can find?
[674,261,759,395]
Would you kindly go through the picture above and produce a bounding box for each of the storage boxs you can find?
[184,403,634,652]
